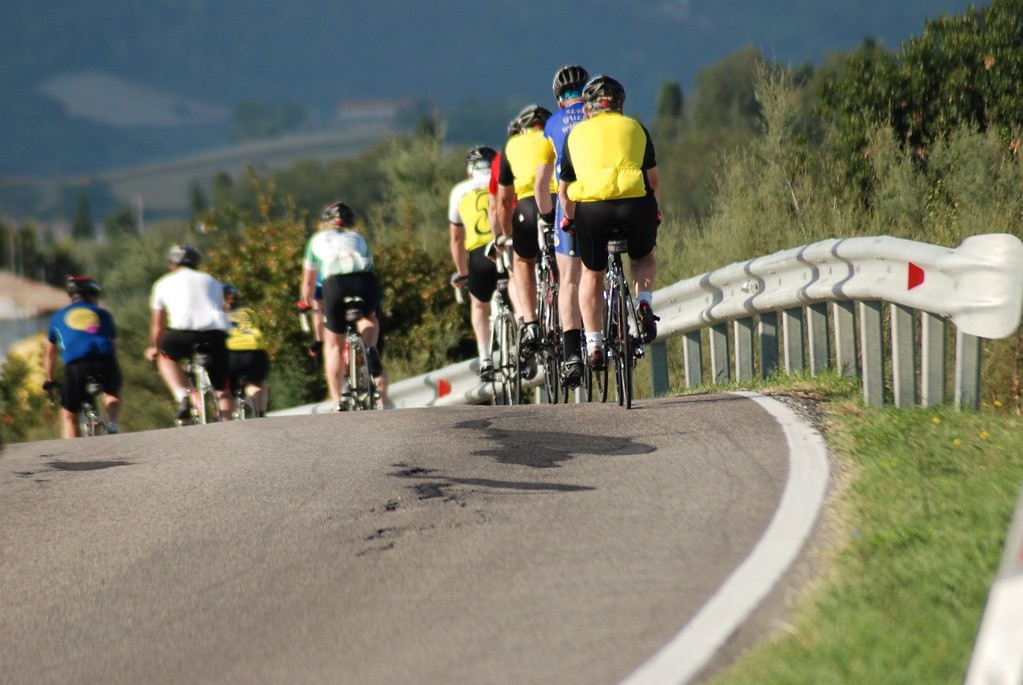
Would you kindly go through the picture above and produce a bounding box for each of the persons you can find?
[447,64,663,387]
[45,276,123,440]
[144,244,233,423]
[216,280,269,421]
[293,202,394,411]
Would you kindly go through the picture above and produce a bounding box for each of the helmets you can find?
[65,275,102,299]
[579,75,626,107]
[321,201,357,232]
[553,64,590,102]
[464,144,497,174]
[507,103,553,140]
[168,244,203,270]
[220,283,238,308]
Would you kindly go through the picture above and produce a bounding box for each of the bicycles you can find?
[153,340,222,427]
[296,294,383,413]
[45,374,119,438]
[230,379,259,421]
[493,224,561,404]
[454,275,523,405]
[562,218,663,409]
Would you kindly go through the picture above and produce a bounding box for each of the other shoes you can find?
[582,345,606,371]
[379,400,393,411]
[518,321,543,349]
[636,300,659,345]
[480,360,492,384]
[367,344,382,379]
[177,398,190,421]
[559,355,582,387]
